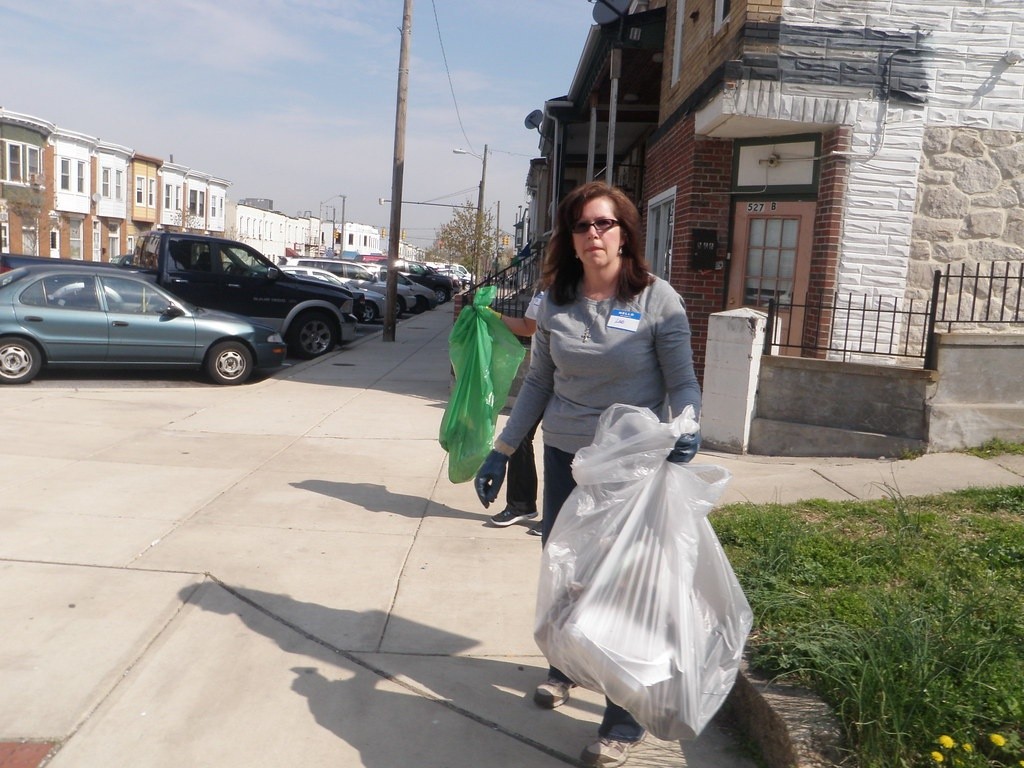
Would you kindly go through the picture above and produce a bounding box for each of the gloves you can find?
[667,432,697,463]
[474,450,509,509]
[485,306,501,320]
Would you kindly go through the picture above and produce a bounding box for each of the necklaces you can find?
[577,289,613,344]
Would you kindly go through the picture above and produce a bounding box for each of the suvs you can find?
[275,256,417,318]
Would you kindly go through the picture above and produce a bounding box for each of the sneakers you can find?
[531,519,542,535]
[580,730,647,768]
[491,506,538,526]
[534,675,578,708]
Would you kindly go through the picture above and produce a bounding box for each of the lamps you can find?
[1002,47,1023,66]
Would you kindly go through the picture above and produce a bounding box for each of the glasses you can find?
[570,219,622,233]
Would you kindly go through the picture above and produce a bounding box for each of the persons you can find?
[480,263,558,537]
[476,181,701,768]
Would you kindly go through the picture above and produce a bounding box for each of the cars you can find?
[251,265,386,324]
[0,264,287,387]
[350,255,475,315]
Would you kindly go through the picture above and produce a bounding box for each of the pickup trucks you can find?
[0,231,361,362]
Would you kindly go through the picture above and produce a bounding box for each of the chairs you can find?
[195,253,221,271]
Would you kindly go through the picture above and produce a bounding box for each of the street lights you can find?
[453,143,488,295]
